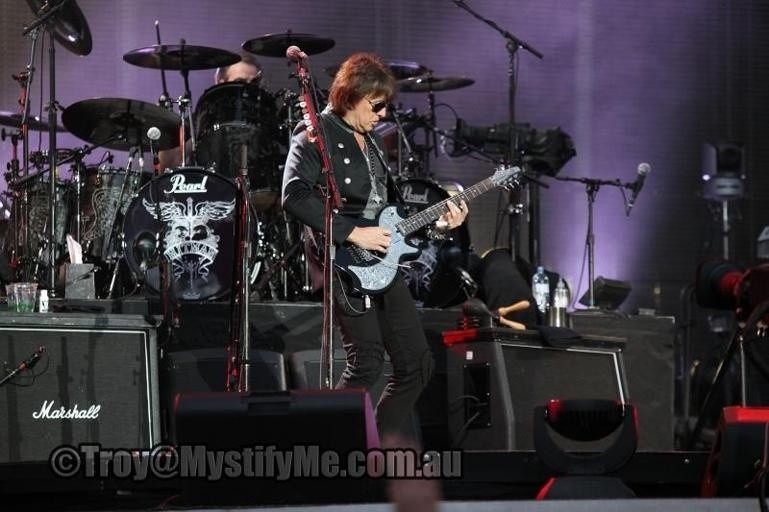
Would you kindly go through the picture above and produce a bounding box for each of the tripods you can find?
[681,313,766,449]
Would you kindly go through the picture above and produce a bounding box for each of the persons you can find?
[281,52,469,462]
[155,56,325,295]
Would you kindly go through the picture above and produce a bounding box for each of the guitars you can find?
[332,164,522,296]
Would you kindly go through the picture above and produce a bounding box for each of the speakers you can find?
[446,340,630,455]
[1,311,163,465]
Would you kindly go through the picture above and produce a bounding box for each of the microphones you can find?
[125,126,165,157]
[624,161,652,217]
[286,46,311,64]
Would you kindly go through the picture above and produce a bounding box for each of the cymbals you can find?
[26,1,91,56]
[0,112,69,134]
[123,44,243,69]
[327,60,429,82]
[60,98,191,153]
[393,76,477,91]
[241,33,334,56]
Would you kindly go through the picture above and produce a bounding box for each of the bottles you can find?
[39,290,49,314]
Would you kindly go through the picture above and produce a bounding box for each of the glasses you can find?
[363,96,390,112]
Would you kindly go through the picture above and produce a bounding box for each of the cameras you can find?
[690,255,768,314]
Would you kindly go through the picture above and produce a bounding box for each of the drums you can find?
[3,176,75,266]
[195,85,282,185]
[280,89,329,149]
[121,168,263,304]
[390,179,470,305]
[70,166,158,261]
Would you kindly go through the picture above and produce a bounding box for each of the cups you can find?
[531,266,550,313]
[14,282,39,314]
[6,283,19,313]
[552,278,569,308]
